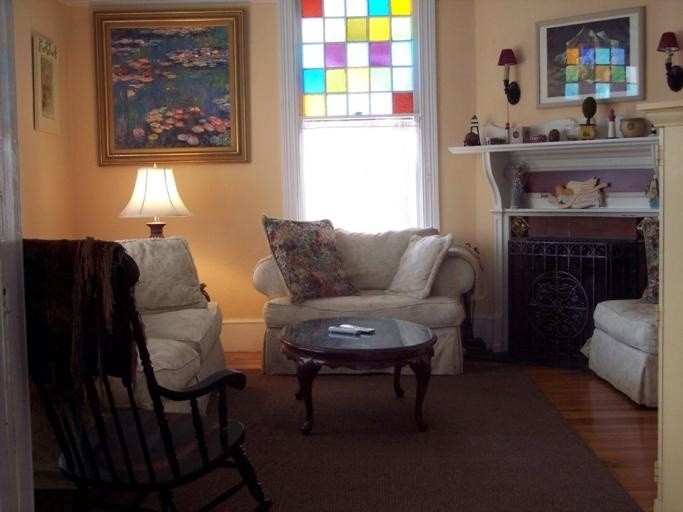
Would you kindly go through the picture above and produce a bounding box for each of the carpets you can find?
[34,362,645,511]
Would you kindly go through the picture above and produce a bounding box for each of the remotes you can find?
[328,326,362,334]
[339,324,376,333]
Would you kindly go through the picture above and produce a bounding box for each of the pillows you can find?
[113,236,208,316]
[261,213,361,305]
[387,234,453,298]
[334,226,439,289]
[637,216,659,304]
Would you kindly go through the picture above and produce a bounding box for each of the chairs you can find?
[23,235,274,511]
[92,280,228,416]
[580,297,657,409]
[252,244,484,376]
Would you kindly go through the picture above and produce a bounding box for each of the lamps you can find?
[117,162,193,237]
[498,49,520,105]
[656,32,683,92]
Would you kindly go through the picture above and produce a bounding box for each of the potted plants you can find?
[577,96,599,139]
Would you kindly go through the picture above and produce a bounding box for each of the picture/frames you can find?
[32,33,60,134]
[92,8,253,164]
[533,3,646,109]
